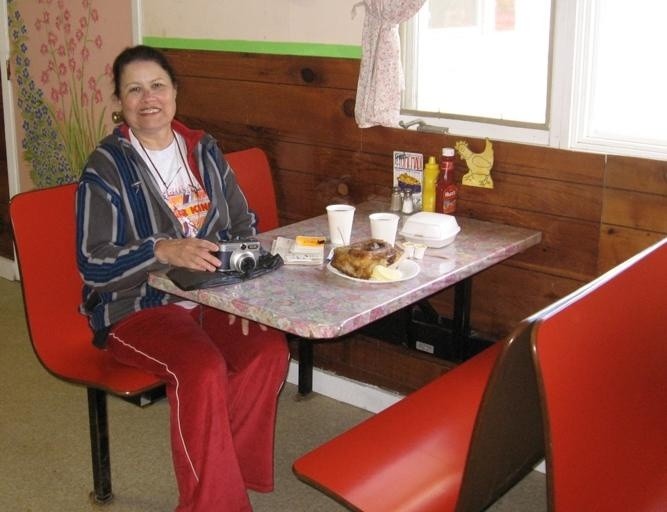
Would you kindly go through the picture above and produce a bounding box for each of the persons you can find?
[75,46,293,510]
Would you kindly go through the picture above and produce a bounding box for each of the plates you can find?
[326,258,421,284]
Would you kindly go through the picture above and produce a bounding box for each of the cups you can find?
[326,204,356,246]
[369,212,400,248]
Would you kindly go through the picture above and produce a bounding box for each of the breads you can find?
[332,239,397,279]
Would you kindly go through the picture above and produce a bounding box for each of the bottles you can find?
[422,155,440,212]
[402,189,415,214]
[390,186,402,212]
[437,147,459,217]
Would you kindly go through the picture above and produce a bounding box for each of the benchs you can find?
[9,145,312,503]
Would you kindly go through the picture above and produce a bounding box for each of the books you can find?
[267,227,326,269]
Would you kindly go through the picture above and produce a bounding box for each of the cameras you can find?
[209,238,261,275]
